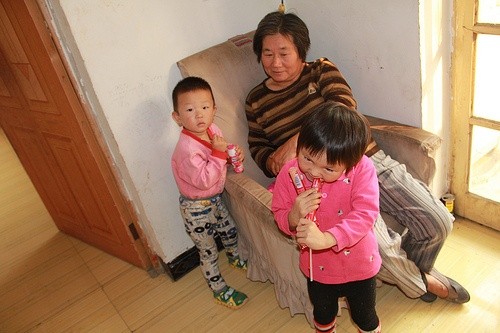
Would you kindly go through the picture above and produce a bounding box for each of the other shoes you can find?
[420,266,470,304]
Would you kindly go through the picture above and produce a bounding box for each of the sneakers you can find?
[229,258,248,270]
[212,284,249,310]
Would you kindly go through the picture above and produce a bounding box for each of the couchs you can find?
[177,30,444,328]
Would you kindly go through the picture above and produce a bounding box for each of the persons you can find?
[245,11,473,310]
[171,74,253,312]
[273,102,391,333]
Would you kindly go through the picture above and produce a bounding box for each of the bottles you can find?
[226,144,245,173]
